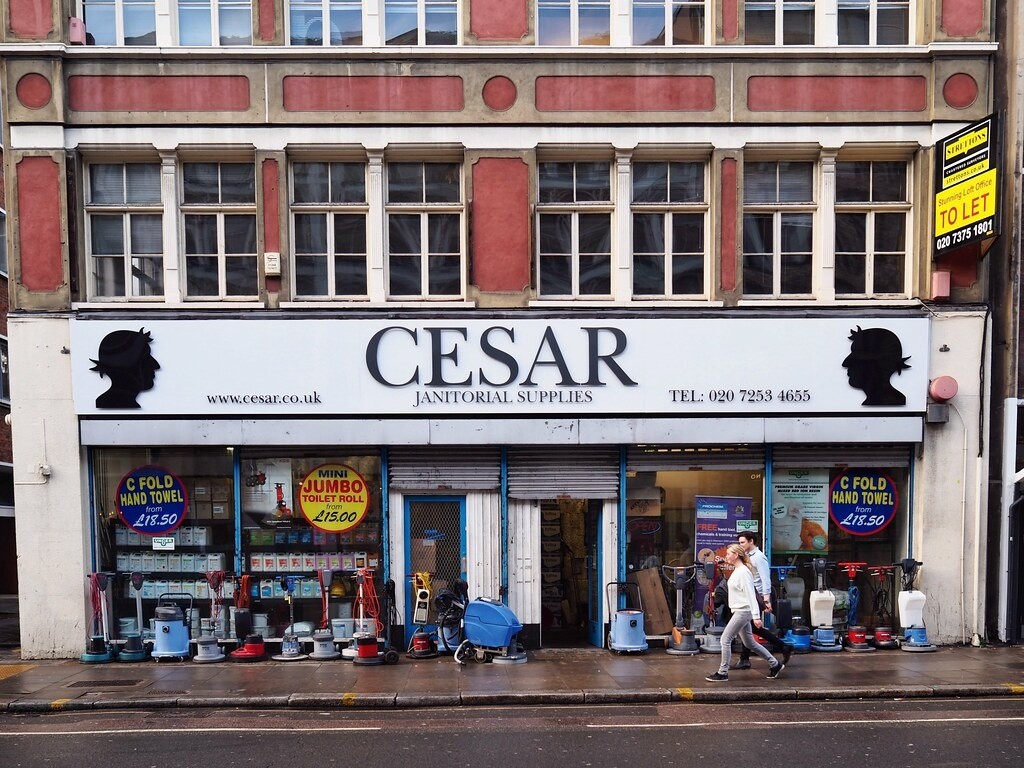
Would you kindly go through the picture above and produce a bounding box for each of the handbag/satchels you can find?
[754,585,767,611]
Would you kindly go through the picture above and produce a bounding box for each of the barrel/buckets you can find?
[118,618,136,632]
[185,608,201,638]
[253,626,269,638]
[253,614,268,626]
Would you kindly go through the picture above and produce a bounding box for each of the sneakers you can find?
[705,672,728,682]
[765,661,785,679]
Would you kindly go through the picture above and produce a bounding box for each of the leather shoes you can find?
[781,644,792,665]
[728,659,751,671]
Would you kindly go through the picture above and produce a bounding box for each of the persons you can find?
[704,544,784,681]
[729,530,792,669]
[628,532,693,570]
